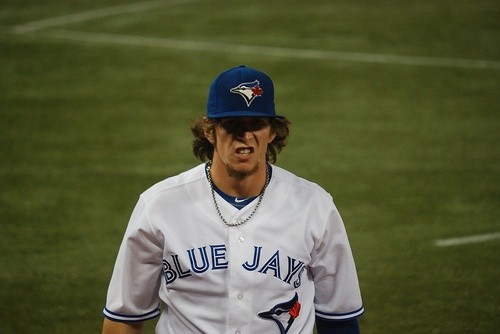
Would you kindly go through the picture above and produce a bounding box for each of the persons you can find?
[101,65,365,334]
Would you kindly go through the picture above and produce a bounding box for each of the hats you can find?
[208,63,276,118]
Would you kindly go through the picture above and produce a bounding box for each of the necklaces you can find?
[207,161,269,227]
